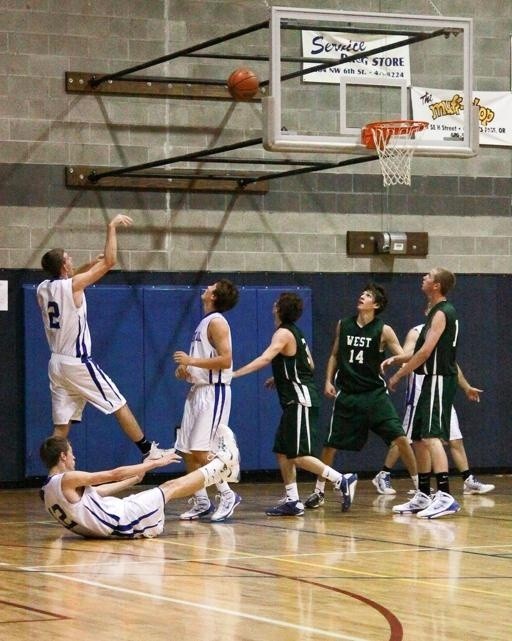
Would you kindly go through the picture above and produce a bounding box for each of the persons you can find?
[304,280,420,509]
[378,267,462,517]
[36,213,177,500]
[231,291,358,516]
[39,423,241,540]
[170,279,243,522]
[370,293,497,495]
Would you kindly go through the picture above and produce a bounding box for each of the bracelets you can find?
[135,475,141,482]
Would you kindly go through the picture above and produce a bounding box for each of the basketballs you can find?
[230,69,259,101]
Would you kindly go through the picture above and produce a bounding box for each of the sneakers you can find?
[180,488,244,520]
[371,468,395,496]
[267,491,327,517]
[391,491,458,519]
[142,441,176,462]
[213,424,241,484]
[335,473,357,512]
[463,475,495,494]
